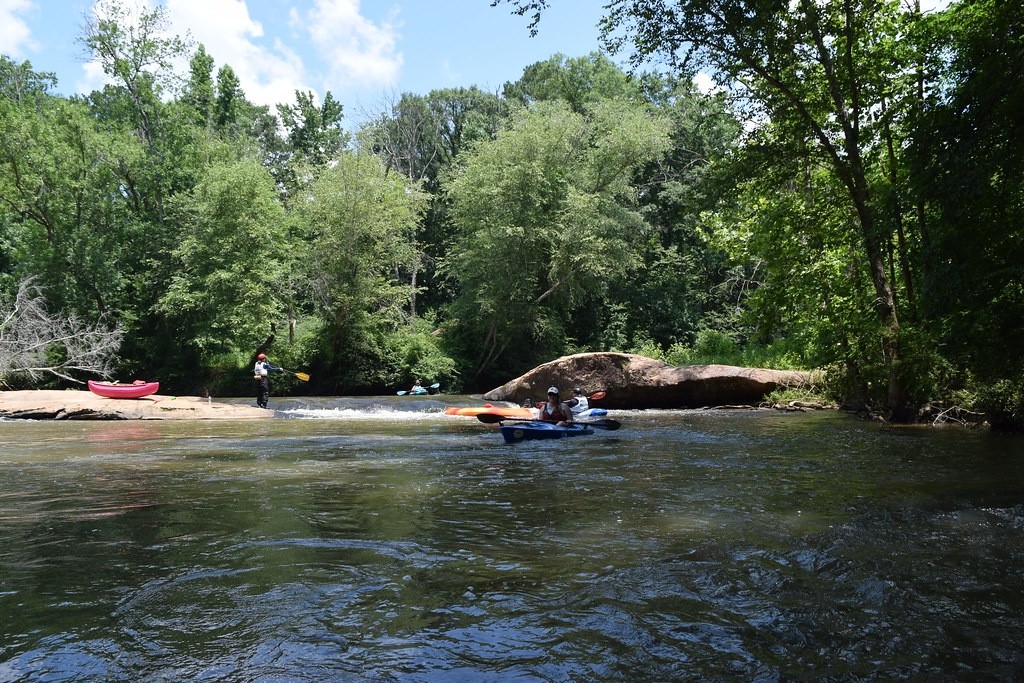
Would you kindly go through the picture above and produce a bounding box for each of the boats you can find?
[409,386,429,394]
[444,407,607,443]
[87,380,160,399]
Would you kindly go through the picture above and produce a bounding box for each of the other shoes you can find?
[260,405,270,409]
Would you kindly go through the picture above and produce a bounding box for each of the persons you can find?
[566,388,588,415]
[411,380,422,391]
[538,387,575,428]
[254,354,283,409]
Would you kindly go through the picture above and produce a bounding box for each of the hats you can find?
[258,353,266,360]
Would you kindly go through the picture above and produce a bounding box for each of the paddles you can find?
[476,413,622,432]
[396,382,440,396]
[535,392,606,409]
[280,367,310,381]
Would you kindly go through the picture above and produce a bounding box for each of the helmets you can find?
[571,388,582,394]
[547,387,560,398]
[416,380,420,385]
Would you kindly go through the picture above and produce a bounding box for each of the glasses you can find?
[548,392,557,397]
